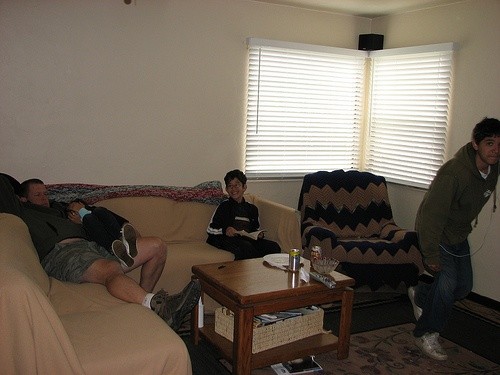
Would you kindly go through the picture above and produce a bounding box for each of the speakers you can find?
[358,33,384,50]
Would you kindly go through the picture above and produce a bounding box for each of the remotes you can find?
[310,271,336,289]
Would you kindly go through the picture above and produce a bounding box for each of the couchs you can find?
[298,164,422,314]
[0,178,305,375]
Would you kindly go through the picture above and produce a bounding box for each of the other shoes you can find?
[111,240,134,268]
[121,223,138,258]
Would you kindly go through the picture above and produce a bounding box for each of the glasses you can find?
[226,184,241,190]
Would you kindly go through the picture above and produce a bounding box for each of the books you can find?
[222,305,319,329]
[270,355,323,375]
[235,229,268,240]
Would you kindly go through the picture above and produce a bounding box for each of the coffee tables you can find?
[189,249,356,375]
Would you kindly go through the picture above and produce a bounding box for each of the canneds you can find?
[288,272,299,289]
[289,248,300,272]
[310,245,322,267]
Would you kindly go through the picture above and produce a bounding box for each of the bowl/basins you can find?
[311,257,340,275]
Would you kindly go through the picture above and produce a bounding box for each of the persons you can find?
[66,199,138,268]
[406,118,500,360]
[0,172,201,332]
[206,170,281,261]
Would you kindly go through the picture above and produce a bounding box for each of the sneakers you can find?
[409,287,423,320]
[150,278,202,331]
[416,332,447,360]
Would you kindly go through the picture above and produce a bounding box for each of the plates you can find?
[263,253,305,266]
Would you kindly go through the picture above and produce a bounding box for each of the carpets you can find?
[216,318,500,375]
